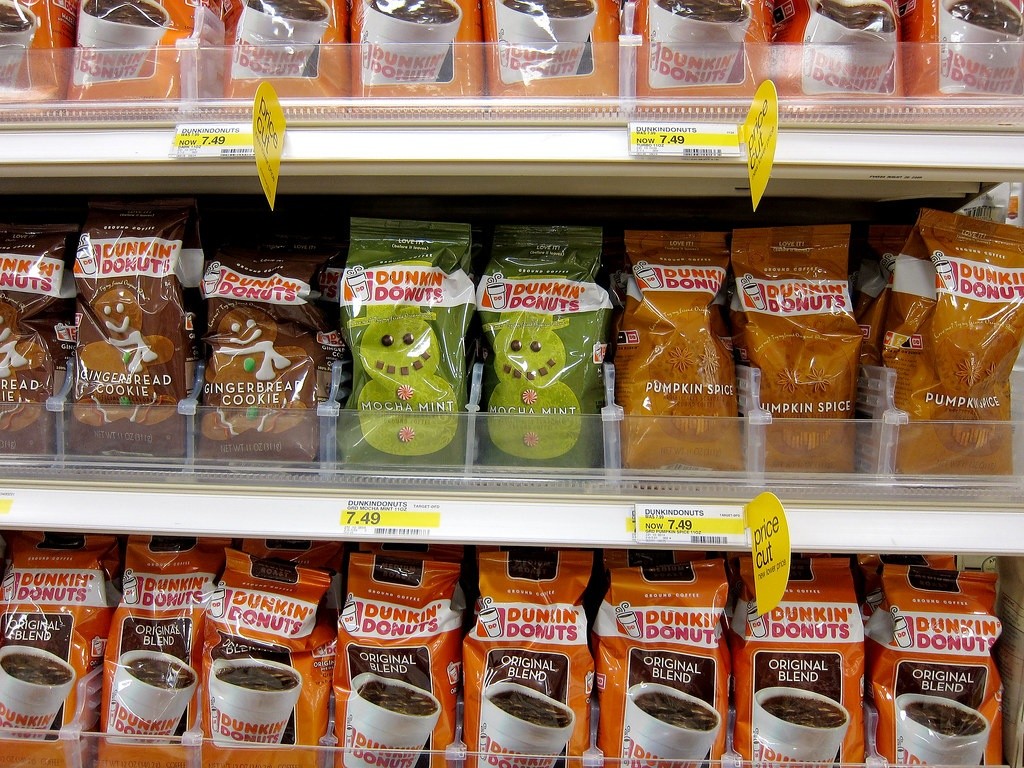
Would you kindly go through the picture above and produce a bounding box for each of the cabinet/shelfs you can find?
[0,0,1024,768]
